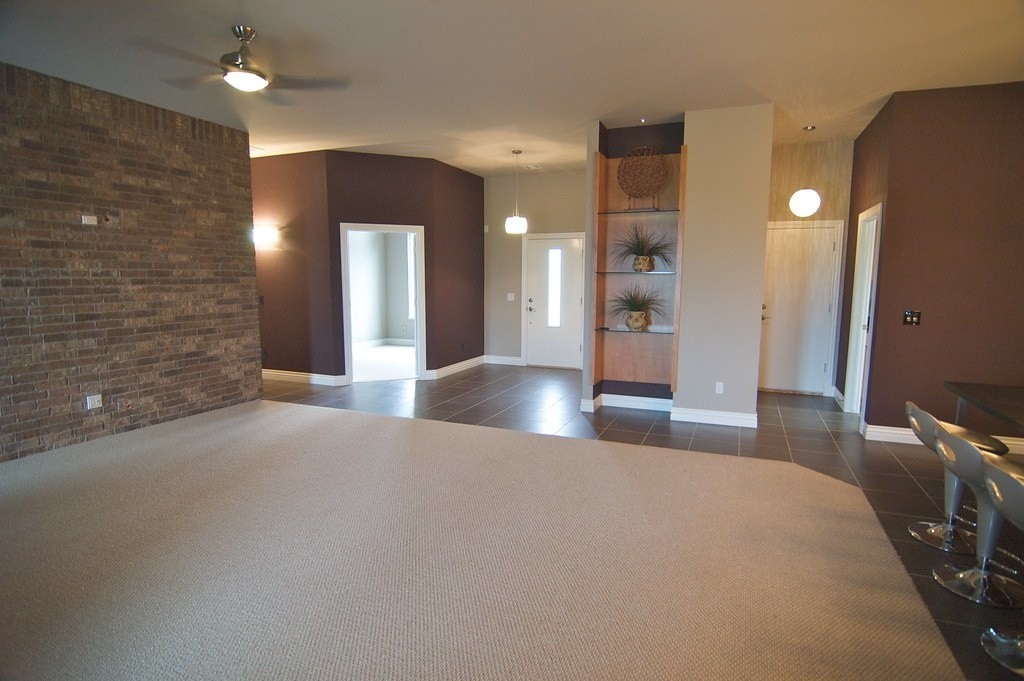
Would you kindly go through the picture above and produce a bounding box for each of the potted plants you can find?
[607,223,676,272]
[605,282,667,331]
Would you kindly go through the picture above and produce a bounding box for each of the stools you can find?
[979,453,1024,678]
[905,399,1010,557]
[933,423,1024,611]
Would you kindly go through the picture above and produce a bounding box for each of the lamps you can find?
[788,125,821,218]
[220,24,269,91]
[504,149,528,235]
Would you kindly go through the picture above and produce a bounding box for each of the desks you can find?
[943,379,1024,431]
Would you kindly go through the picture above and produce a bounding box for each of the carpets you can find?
[0,398,967,681]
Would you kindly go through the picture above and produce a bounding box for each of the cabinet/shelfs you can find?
[593,209,679,335]
